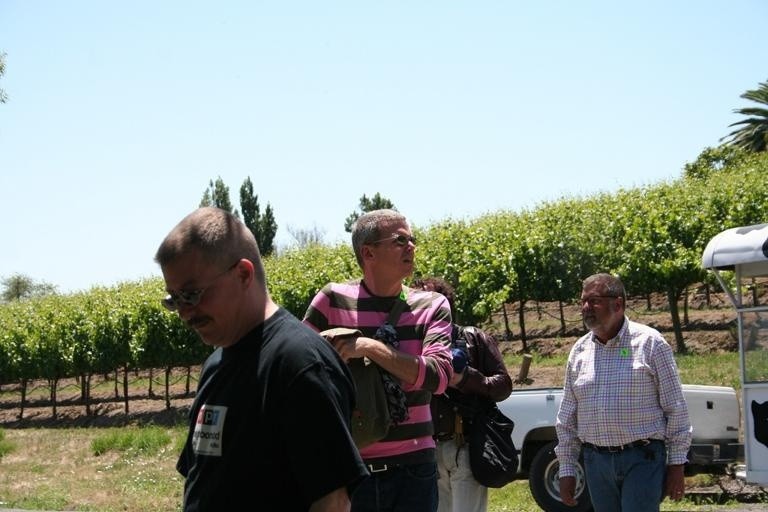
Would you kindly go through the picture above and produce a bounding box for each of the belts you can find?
[581,438,653,453]
[362,447,437,473]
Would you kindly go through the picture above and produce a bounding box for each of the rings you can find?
[677,491,682,494]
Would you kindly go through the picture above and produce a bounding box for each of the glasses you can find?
[370,236,418,248]
[577,295,616,306]
[160,263,235,313]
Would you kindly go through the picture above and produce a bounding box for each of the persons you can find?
[301,209,453,512]
[411,278,512,512]
[553,273,693,512]
[153,207,371,512]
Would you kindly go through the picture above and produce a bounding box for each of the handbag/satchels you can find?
[464,403,519,488]
[318,327,391,451]
